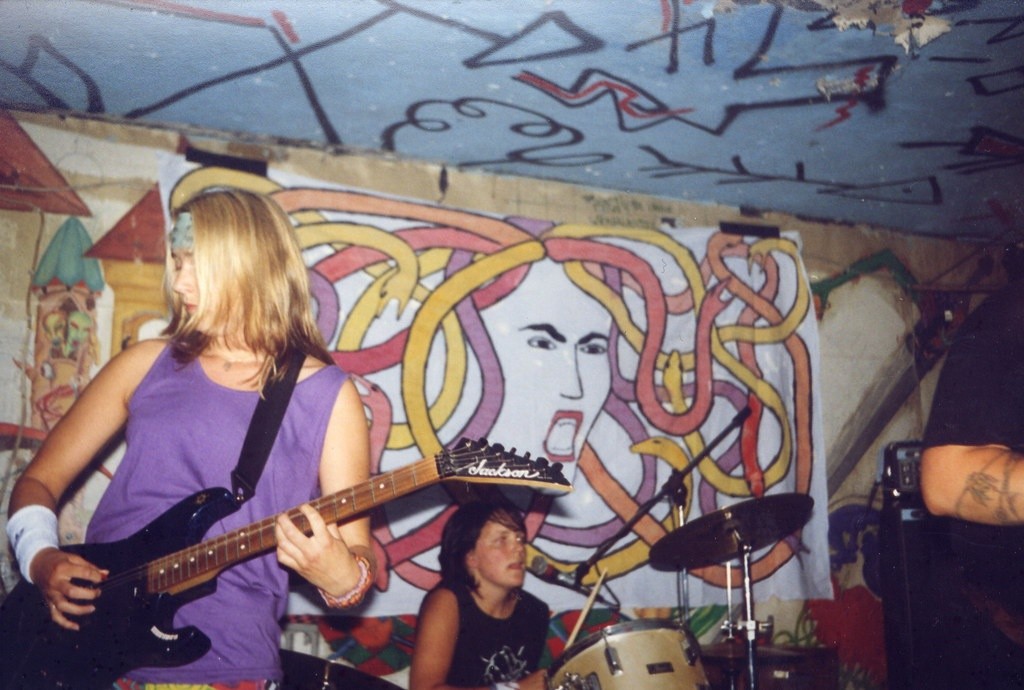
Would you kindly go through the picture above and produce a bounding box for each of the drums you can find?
[547,617,710,690]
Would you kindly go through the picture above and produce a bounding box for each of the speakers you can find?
[882,497,1024,690]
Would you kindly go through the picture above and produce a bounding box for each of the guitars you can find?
[0,437,572,690]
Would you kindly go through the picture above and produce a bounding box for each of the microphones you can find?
[531,556,606,602]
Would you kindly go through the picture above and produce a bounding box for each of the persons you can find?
[7,184,378,690]
[920,281,1024,690]
[409,499,549,690]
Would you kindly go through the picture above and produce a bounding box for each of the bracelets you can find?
[5,504,60,584]
[317,552,373,609]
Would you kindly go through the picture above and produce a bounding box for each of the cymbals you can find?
[649,492,815,572]
[698,643,803,659]
